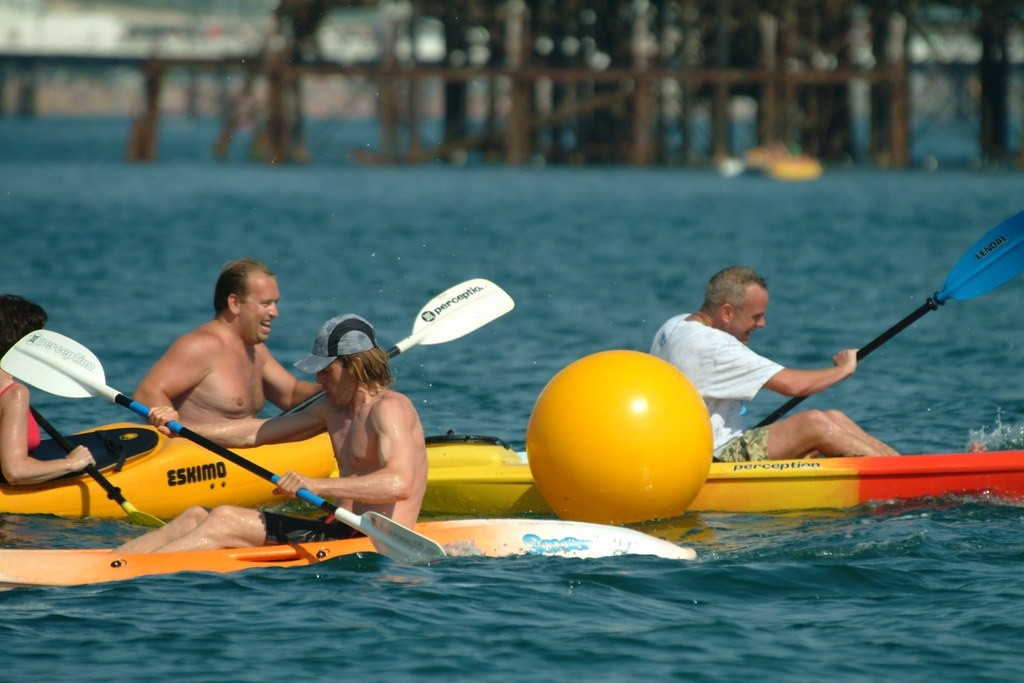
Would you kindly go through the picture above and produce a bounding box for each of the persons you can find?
[105,312,427,553]
[647,266,990,461]
[129,258,325,448]
[0,291,97,484]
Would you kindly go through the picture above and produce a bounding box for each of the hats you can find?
[292,313,377,374]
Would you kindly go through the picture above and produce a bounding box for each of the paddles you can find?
[275,278,516,420]
[0,329,447,560]
[752,211,1024,428]
[29,405,166,529]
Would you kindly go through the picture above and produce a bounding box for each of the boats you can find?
[0,518,698,587]
[721,158,822,181]
[0,423,340,517]
[421,435,1024,514]
